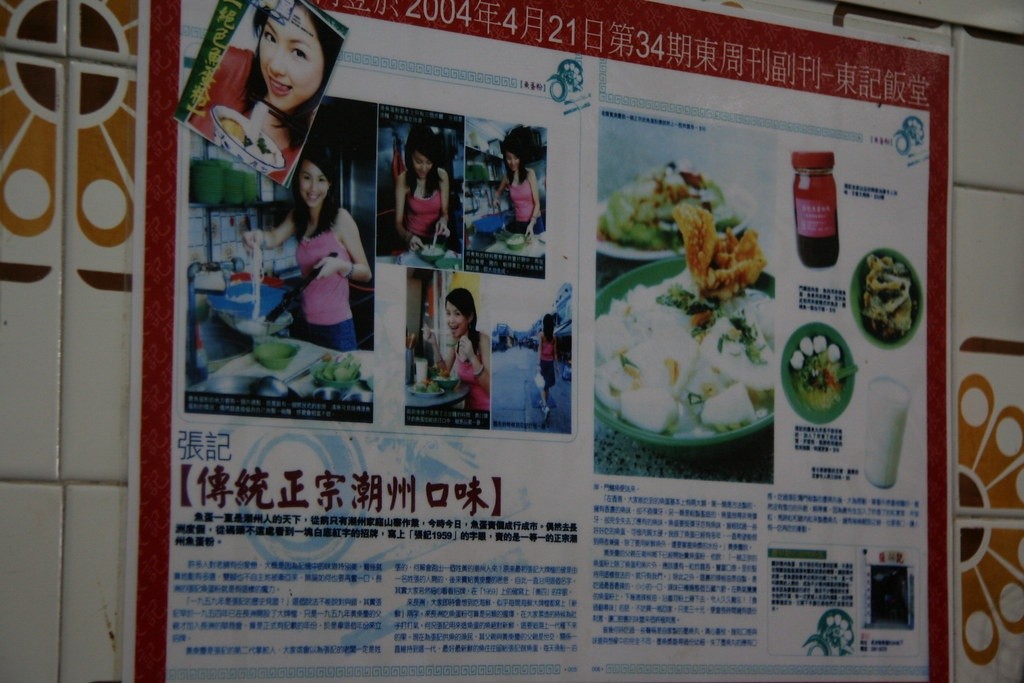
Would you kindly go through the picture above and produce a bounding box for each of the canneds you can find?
[791,150,841,269]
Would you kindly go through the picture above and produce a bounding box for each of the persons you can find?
[493,143,544,243]
[537,313,561,421]
[189,0,345,186]
[424,288,490,411]
[241,153,371,350]
[396,124,460,254]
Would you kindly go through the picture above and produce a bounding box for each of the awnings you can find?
[530,322,571,340]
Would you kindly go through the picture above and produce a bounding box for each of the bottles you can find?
[790,150,840,270]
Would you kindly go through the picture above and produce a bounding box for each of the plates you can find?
[596,199,748,261]
[435,258,462,270]
[407,387,444,397]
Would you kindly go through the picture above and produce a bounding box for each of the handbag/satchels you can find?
[535,367,546,389]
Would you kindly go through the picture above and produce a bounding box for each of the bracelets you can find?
[345,263,353,278]
[474,365,484,378]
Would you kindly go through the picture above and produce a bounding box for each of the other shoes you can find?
[540,400,550,421]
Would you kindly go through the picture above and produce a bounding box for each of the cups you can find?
[859,376,915,488]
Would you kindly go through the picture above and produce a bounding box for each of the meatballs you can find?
[791,335,841,368]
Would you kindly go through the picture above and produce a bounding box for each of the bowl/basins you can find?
[253,342,298,369]
[317,364,362,389]
[595,257,775,462]
[207,104,287,175]
[849,246,923,350]
[780,321,856,424]
[505,234,526,251]
[189,158,257,204]
[433,378,460,389]
[416,244,446,263]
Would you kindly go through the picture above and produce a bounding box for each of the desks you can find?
[405,374,471,426]
